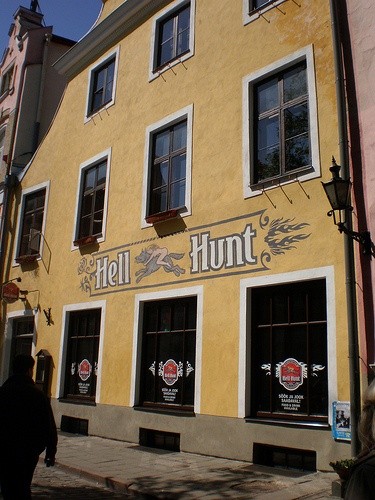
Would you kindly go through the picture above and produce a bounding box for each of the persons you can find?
[342,398,375,500]
[0,355,59,500]
